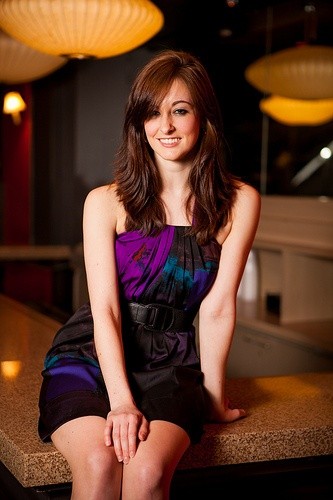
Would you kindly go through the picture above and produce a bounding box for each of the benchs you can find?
[0,291,333,499]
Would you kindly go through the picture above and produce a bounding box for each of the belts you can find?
[121,302,196,333]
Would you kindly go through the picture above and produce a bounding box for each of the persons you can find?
[36,51,263,500]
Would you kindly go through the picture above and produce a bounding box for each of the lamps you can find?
[0,30,67,83]
[258,92,333,126]
[0,0,165,63]
[3,91,27,126]
[243,2,333,100]
